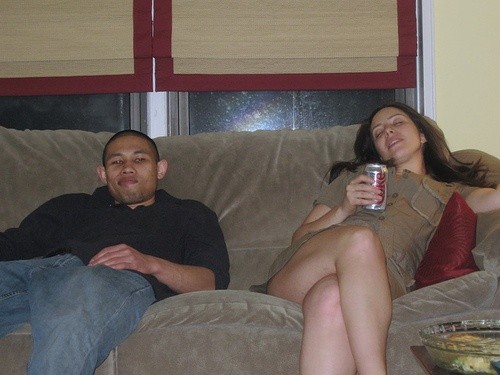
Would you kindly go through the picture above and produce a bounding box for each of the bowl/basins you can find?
[419,320,500,374]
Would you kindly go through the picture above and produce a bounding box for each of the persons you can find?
[0,129,230,375]
[248,100,500,375]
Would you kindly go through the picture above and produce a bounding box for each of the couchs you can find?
[0,117,500,375]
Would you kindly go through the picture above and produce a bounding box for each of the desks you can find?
[411,345,462,375]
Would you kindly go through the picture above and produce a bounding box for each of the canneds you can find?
[364,164,387,210]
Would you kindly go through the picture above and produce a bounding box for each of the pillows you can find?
[416,191,479,288]
